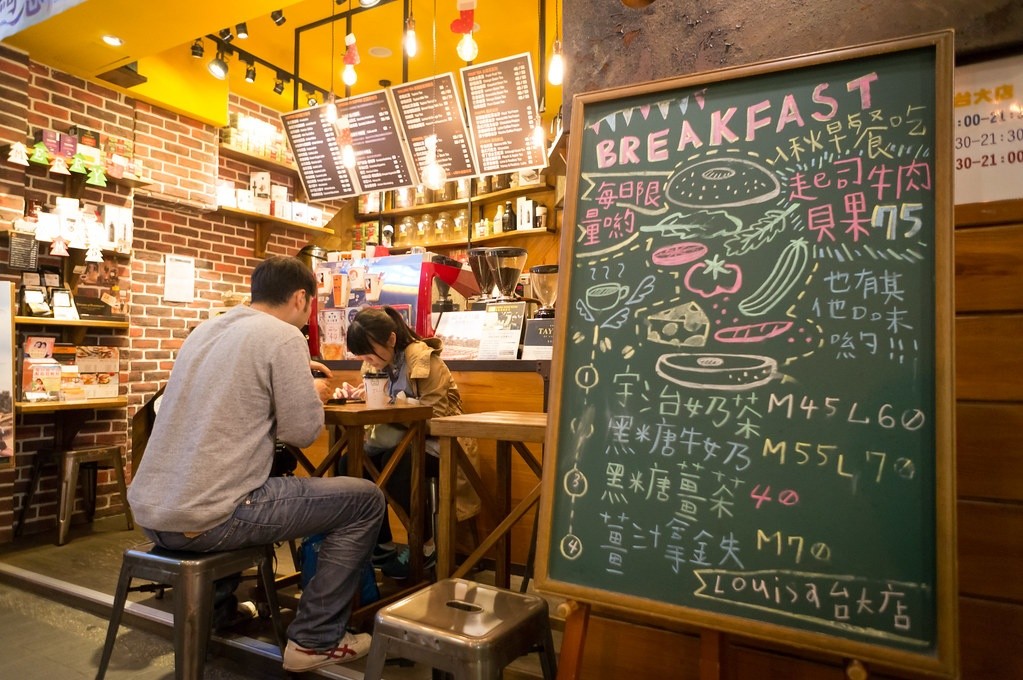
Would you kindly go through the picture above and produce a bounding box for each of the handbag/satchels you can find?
[298,531,379,608]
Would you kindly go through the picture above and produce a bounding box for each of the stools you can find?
[94,544,288,680]
[363,577,557,680]
[16,442,133,547]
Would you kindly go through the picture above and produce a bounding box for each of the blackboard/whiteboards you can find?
[535,27,959,677]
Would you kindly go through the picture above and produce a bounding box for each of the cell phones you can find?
[346,400,366,403]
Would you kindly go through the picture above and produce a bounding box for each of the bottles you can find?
[397,173,548,247]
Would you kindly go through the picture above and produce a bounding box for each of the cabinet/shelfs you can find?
[214,142,335,257]
[2,144,153,449]
[355,183,556,255]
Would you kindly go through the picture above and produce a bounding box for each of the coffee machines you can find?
[484,246,528,302]
[530,264,559,318]
[466,247,496,304]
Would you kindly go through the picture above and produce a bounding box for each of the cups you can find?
[327,252,341,262]
[375,245,389,257]
[363,273,384,302]
[311,371,328,383]
[363,371,390,409]
[351,249,361,260]
[331,274,351,309]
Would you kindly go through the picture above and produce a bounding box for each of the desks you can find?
[429,410,548,582]
[254,400,435,633]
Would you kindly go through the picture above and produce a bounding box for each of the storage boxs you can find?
[35,124,143,176]
[21,344,120,403]
[235,171,324,228]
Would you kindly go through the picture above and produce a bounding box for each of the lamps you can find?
[190,0,345,107]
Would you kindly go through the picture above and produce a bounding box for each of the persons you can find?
[334,305,482,580]
[126,255,383,673]
[34,341,46,349]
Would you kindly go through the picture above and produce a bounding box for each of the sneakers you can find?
[212,601,255,632]
[381,547,437,579]
[283,630,372,672]
[372,546,397,560]
